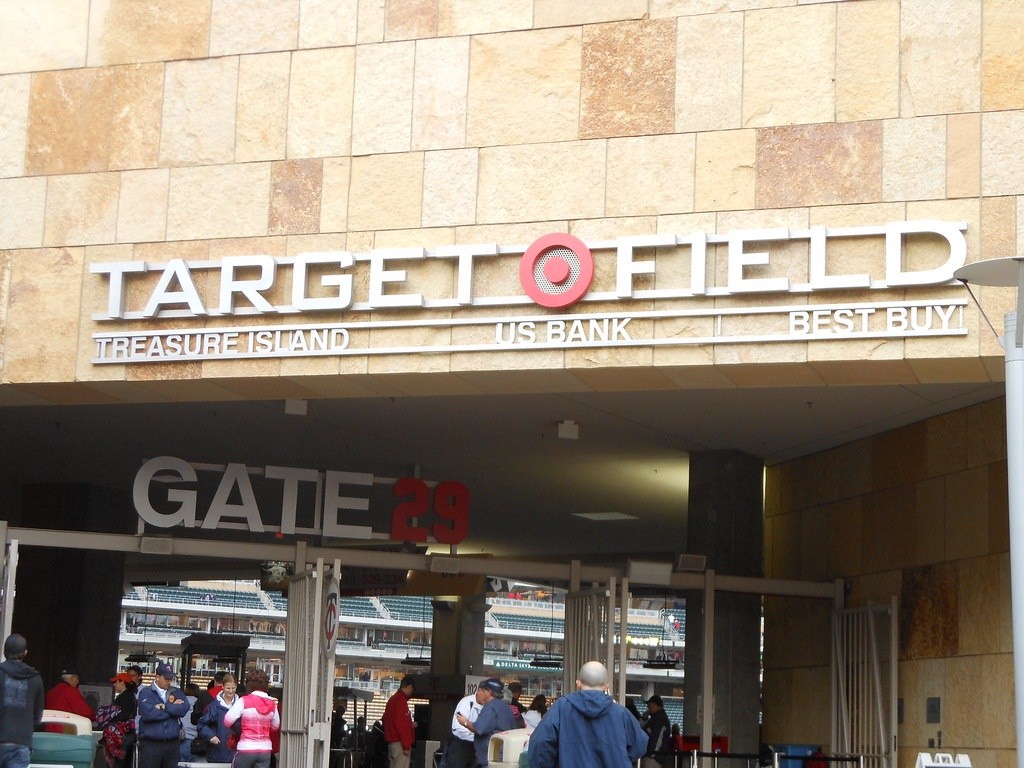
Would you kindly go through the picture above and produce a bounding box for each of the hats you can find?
[59,665,79,676]
[111,674,132,684]
[154,663,177,679]
[644,693,661,704]
[480,679,504,692]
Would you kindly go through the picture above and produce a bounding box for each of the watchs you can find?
[464,718,469,727]
[159,703,163,709]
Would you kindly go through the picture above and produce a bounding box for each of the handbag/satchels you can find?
[179,729,186,740]
[190,736,209,756]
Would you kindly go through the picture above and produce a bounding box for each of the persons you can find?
[528,661,649,768]
[126,663,282,768]
[362,672,370,682]
[329,704,390,768]
[674,620,680,630]
[625,694,683,768]
[94,673,138,768]
[383,677,418,768]
[444,677,548,768]
[44,667,95,733]
[668,614,675,625]
[0,634,44,768]
